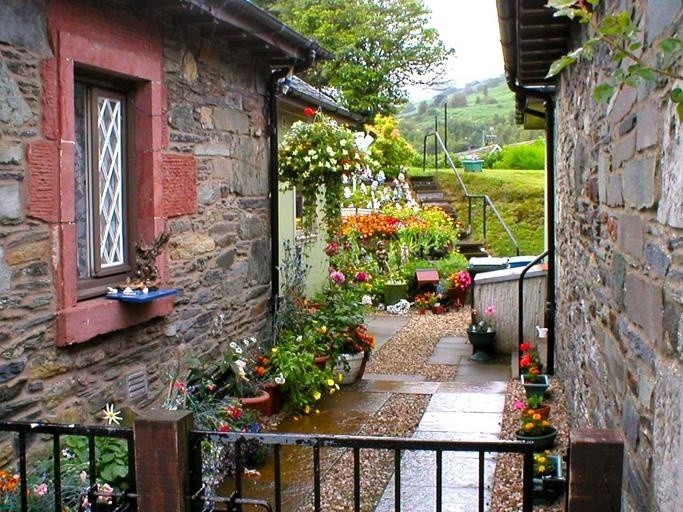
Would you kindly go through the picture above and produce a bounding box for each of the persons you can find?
[375,241,390,274]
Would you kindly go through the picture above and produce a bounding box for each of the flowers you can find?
[215,338,286,396]
[3,403,123,511]
[270,295,373,354]
[270,337,343,415]
[467,305,497,332]
[511,337,564,507]
[167,373,260,510]
[284,120,471,317]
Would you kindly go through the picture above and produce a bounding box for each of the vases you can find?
[310,350,369,385]
[465,328,497,362]
[239,381,281,416]
